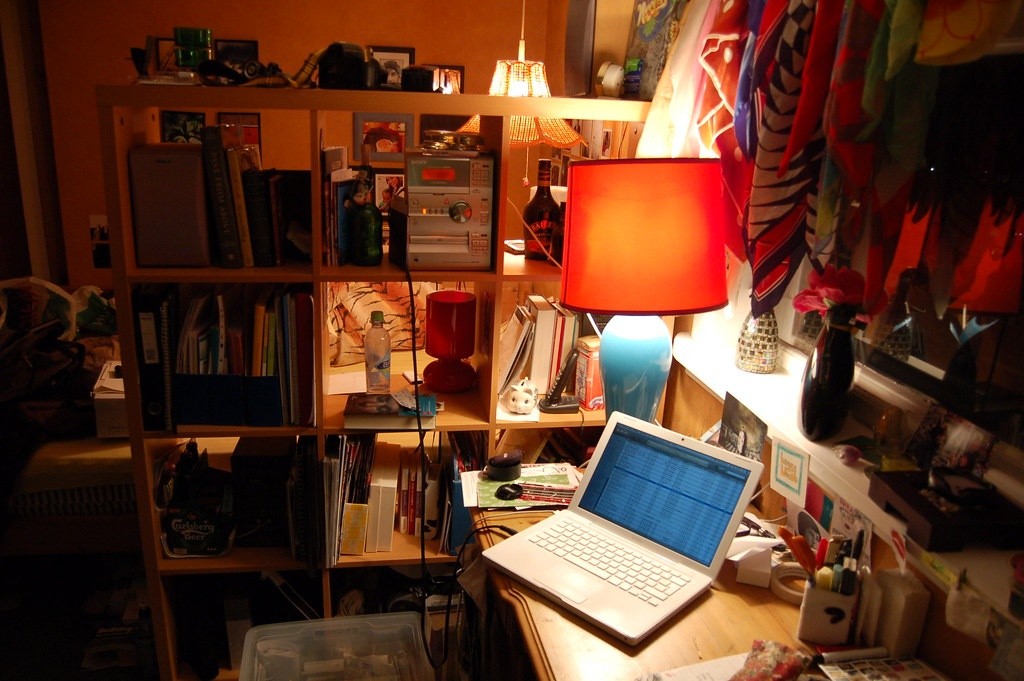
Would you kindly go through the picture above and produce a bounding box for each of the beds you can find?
[0,436,242,554]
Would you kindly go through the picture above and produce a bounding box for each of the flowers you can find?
[791,264,872,384]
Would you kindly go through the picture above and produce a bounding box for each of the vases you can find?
[738,310,780,373]
[799,313,854,442]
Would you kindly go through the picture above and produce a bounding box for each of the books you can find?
[327,433,485,566]
[138,289,317,429]
[322,145,377,268]
[501,293,612,413]
[129,126,315,272]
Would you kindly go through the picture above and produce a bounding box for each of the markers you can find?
[812,646,889,663]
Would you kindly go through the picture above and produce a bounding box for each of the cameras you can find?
[318,42,383,90]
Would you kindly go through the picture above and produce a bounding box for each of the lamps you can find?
[560,158,731,420]
[454,0,584,187]
[424,291,476,394]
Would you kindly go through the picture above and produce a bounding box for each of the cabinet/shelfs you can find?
[93,81,675,681]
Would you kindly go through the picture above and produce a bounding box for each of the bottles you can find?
[524,158,561,268]
[365,312,390,394]
[352,166,383,266]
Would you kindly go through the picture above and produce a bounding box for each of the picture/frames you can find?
[214,39,258,64]
[367,45,415,90]
[160,110,206,145]
[422,64,465,94]
[217,112,262,165]
[353,110,414,162]
[155,37,176,71]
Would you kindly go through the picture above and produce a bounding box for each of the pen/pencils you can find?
[742,516,776,539]
[814,529,865,596]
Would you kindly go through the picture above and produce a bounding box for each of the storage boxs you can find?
[93,360,128,439]
[340,503,368,555]
[366,442,401,552]
[239,611,436,681]
[224,597,251,670]
[575,335,604,412]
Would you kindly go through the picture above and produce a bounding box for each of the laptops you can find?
[479,409,765,647]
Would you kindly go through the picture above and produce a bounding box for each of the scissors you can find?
[777,525,817,585]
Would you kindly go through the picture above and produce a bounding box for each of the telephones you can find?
[538,349,579,414]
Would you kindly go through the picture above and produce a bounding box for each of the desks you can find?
[470,468,833,681]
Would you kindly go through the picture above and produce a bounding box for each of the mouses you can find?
[495,484,524,500]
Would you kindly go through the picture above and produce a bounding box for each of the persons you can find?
[737,430,747,456]
[382,176,404,207]
[385,61,401,88]
[798,511,821,549]
